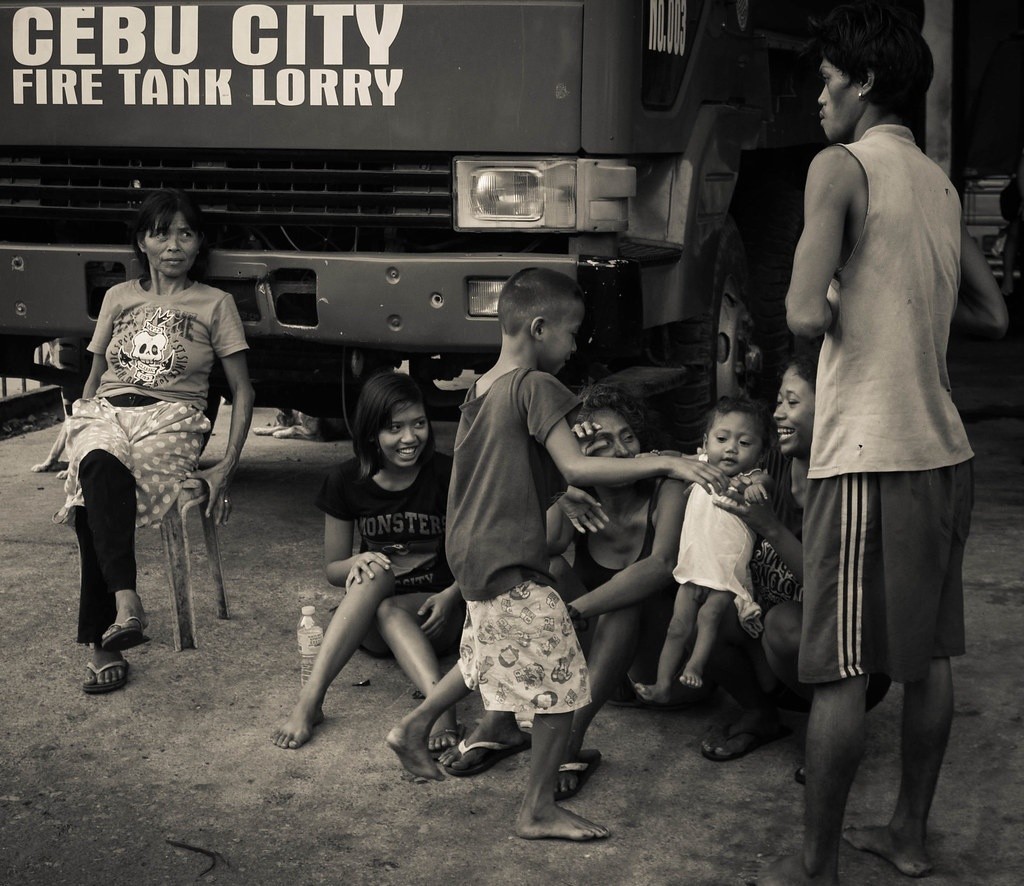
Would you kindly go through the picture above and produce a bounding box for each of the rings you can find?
[225,500,229,503]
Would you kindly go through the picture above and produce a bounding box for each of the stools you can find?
[149,469,233,653]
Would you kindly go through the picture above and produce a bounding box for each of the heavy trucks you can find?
[0,0,1024,463]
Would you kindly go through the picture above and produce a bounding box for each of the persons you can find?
[434,382,688,799]
[51,191,257,692]
[268,371,455,752]
[697,352,817,785]
[758,18,1010,886]
[382,266,731,842]
[631,391,769,707]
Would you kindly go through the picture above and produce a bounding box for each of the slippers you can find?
[552,749,601,801]
[82,659,127,691]
[700,716,794,761]
[428,720,464,759]
[443,731,532,777]
[795,763,806,787]
[102,617,151,651]
